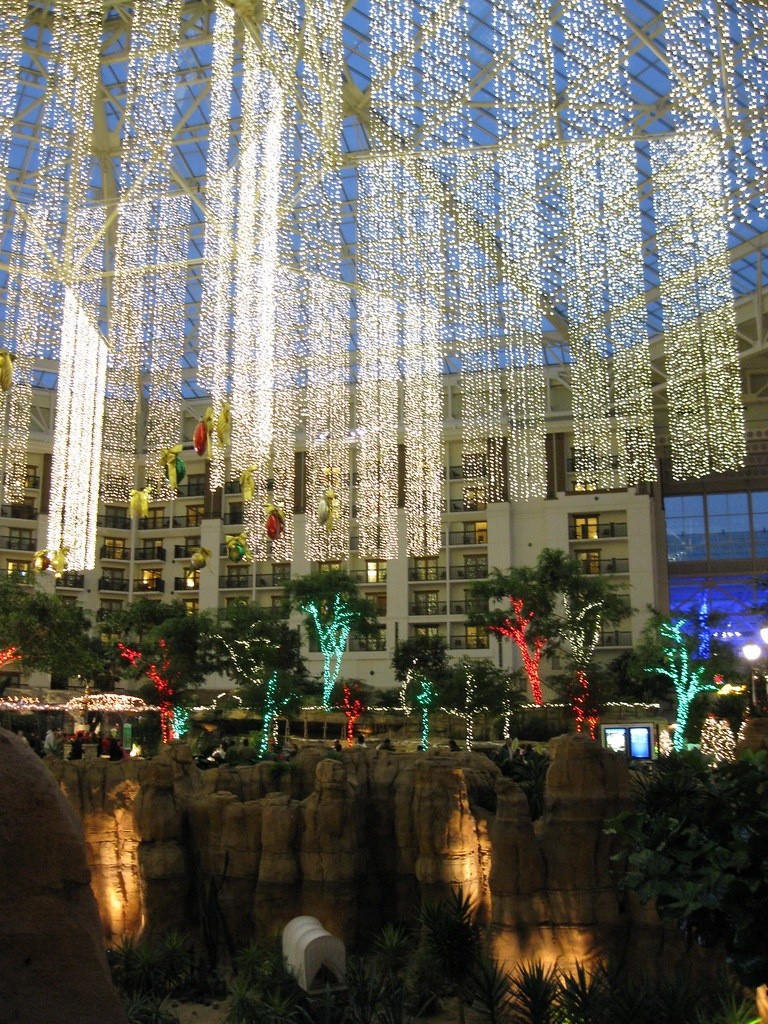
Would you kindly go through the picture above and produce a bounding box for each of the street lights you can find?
[741,625,768,707]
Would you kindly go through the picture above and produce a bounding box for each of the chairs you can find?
[602,529,609,535]
[606,565,612,572]
[439,470,465,647]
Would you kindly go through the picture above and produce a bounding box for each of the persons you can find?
[17,726,534,769]
